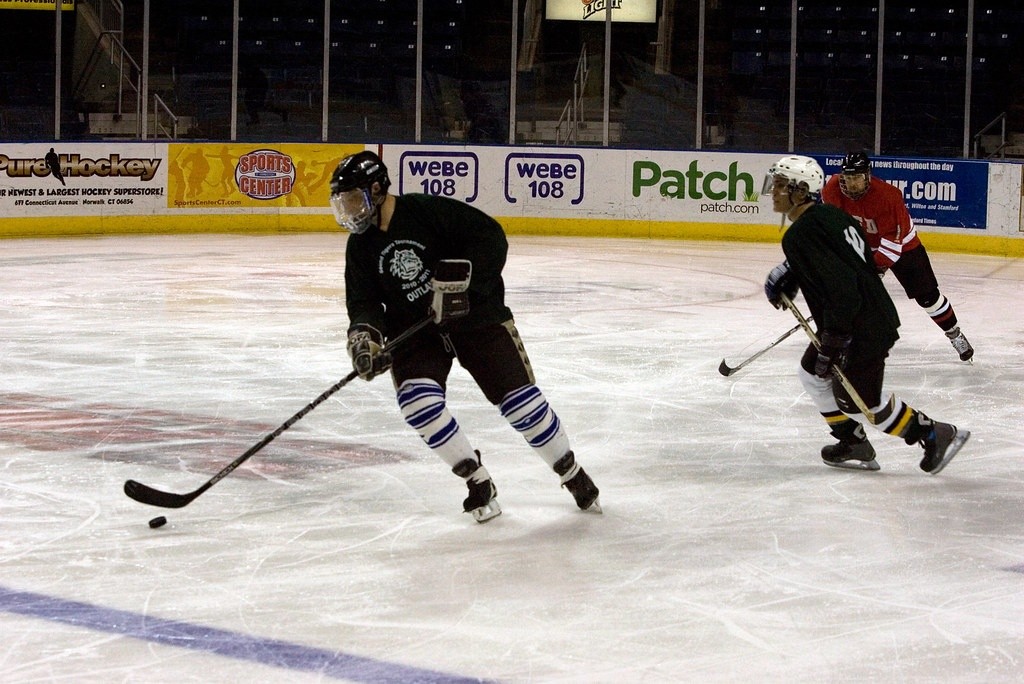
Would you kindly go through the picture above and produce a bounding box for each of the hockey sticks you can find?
[782,295,896,426]
[124,315,431,510]
[718,315,814,377]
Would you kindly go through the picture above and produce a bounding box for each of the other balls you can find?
[149,515,167,529]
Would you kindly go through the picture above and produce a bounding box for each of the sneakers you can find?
[553,451,605,515]
[451,449,503,524]
[821,423,880,470]
[906,409,971,477]
[945,329,974,366]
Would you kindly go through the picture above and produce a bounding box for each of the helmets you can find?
[765,155,825,202]
[839,150,872,201]
[329,150,392,234]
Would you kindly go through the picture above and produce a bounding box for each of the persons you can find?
[764,155,971,476]
[822,155,975,365]
[329,151,603,523]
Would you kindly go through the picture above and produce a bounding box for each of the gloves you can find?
[346,331,392,381]
[428,260,472,327]
[765,258,802,311]
[815,330,854,380]
